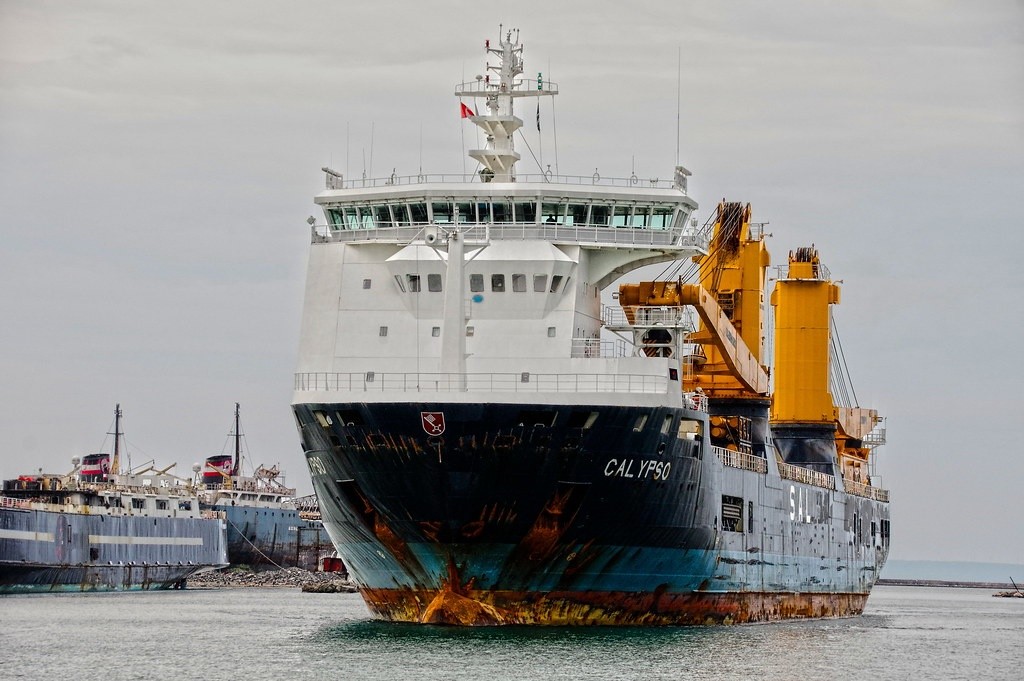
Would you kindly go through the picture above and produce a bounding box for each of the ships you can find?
[291,19,892,629]
[0,470,231,592]
[74,398,339,570]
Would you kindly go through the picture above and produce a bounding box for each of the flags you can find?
[460,102,475,118]
